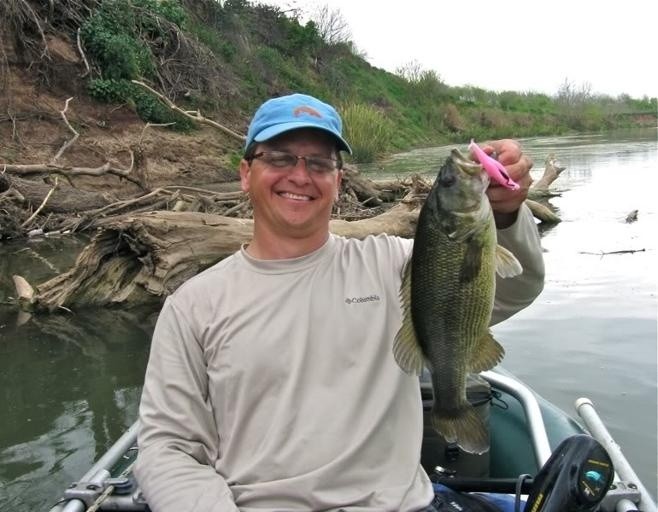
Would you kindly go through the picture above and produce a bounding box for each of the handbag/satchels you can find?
[420,368,492,482]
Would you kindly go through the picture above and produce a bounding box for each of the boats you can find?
[49,348,656,512]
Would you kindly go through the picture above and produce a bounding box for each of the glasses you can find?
[251,150,341,174]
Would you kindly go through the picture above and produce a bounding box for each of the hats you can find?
[245,93,354,156]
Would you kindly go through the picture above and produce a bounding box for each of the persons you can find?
[129,93,549,512]
